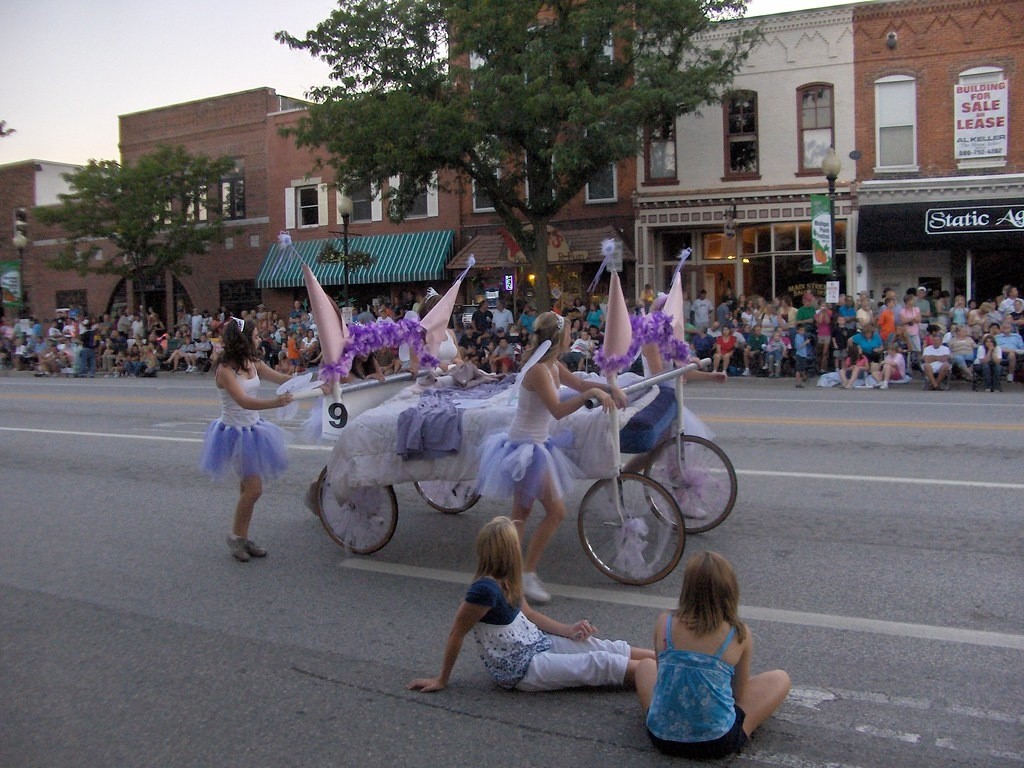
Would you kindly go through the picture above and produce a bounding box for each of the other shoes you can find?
[225,533,251,561]
[795,384,805,388]
[190,365,199,373]
[742,370,750,376]
[678,502,707,519]
[185,366,192,372]
[247,539,267,557]
[880,384,889,390]
[1007,373,1014,382]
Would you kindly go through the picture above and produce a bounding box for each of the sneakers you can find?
[520,570,550,602]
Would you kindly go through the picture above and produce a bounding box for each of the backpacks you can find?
[88,328,101,348]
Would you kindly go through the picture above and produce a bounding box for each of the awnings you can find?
[255,229,456,288]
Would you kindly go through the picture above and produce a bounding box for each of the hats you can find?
[256,303,267,309]
[293,314,301,319]
[278,326,286,332]
[203,309,208,313]
[917,286,927,291]
[83,319,89,324]
[804,295,815,302]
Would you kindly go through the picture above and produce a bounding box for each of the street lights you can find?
[822,148,842,328]
[14,230,28,348]
[329,195,362,307]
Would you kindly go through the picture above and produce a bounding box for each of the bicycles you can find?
[289,362,738,587]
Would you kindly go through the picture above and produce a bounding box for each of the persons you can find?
[635,551,790,760]
[641,292,728,518]
[211,316,331,562]
[405,516,657,692]
[0,283,1024,393]
[507,311,628,602]
[409,287,464,384]
